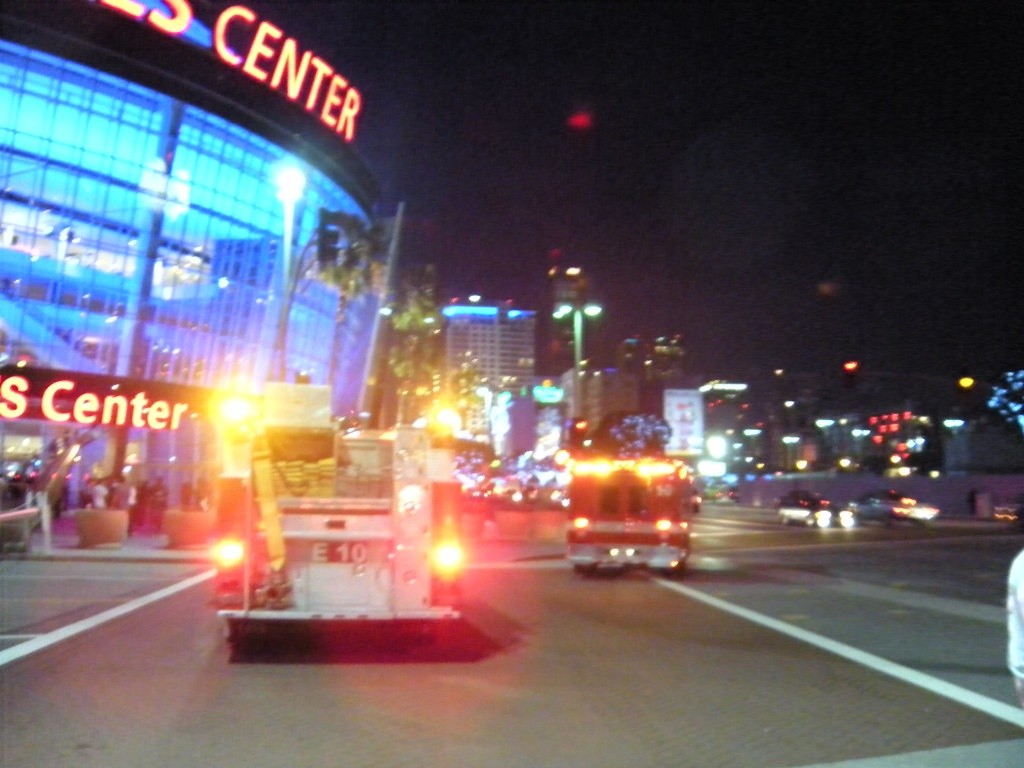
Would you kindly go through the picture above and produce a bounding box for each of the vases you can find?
[75,509,128,551]
[162,509,216,552]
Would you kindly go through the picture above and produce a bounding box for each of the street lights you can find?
[552,301,603,420]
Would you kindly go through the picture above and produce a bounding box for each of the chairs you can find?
[0,440,80,559]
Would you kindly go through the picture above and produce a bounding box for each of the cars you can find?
[775,488,943,529]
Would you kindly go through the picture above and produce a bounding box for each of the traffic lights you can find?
[569,415,589,448]
[314,207,341,262]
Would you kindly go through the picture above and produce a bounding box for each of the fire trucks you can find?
[553,447,702,577]
[211,377,467,659]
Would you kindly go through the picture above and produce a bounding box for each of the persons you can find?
[0,470,213,532]
[1006,548,1024,696]
[92,477,109,508]
